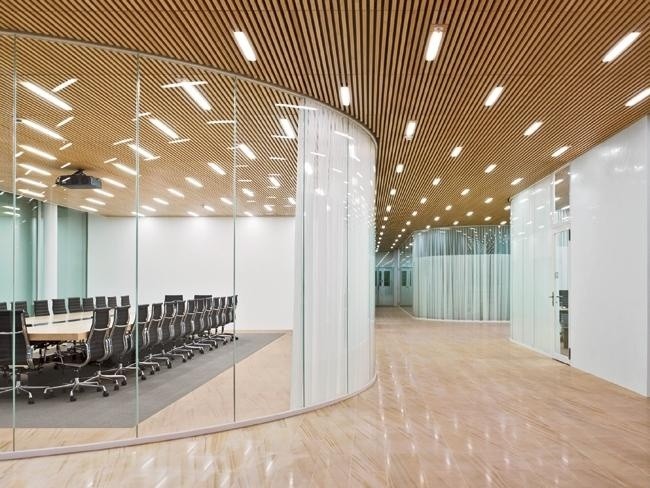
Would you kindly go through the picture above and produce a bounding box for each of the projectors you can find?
[56,175,102,189]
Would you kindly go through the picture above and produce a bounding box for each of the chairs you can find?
[1,291,239,401]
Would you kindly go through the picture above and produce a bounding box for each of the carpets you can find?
[1,329,283,433]
